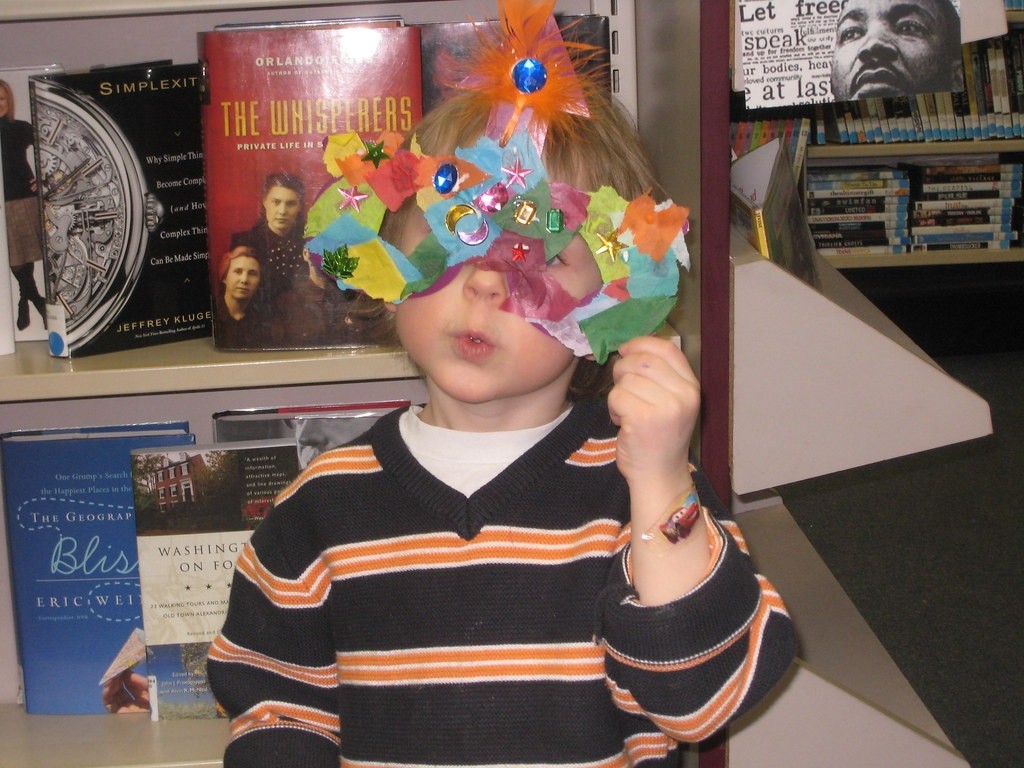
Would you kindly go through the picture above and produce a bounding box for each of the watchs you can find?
[30,77,164,354]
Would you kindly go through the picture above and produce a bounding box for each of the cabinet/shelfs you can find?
[732,10,1024,268]
[0,0,730,768]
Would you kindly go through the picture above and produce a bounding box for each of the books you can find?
[731,0,1024,256]
[0,13,612,358]
[0,399,411,723]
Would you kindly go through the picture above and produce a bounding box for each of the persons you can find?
[273,247,333,347]
[831,0,961,101]
[214,247,274,349]
[1,79,47,331]
[207,76,799,767]
[230,172,304,308]
[102,672,150,713]
